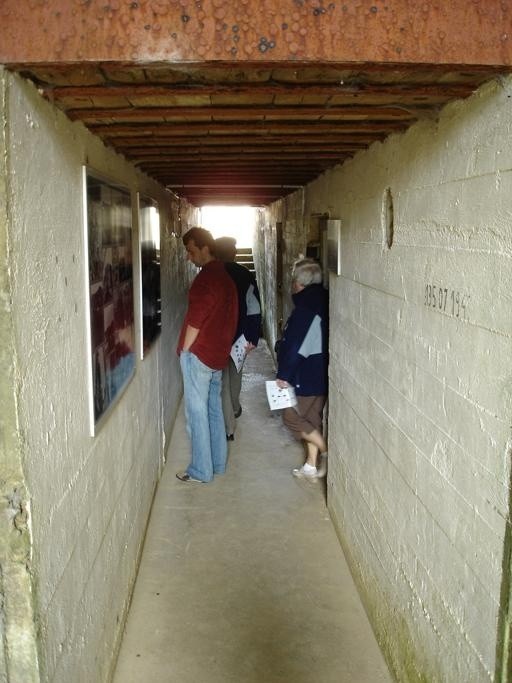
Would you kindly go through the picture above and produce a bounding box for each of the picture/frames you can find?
[78,165,161,438]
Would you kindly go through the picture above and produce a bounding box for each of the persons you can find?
[173,225,241,485]
[288,258,315,280]
[273,258,327,485]
[214,234,263,442]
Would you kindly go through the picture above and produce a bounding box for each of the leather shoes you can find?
[235,408,242,417]
[226,434,234,441]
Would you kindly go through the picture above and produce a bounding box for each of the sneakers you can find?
[176,472,210,483]
[317,452,328,478]
[292,465,317,483]
